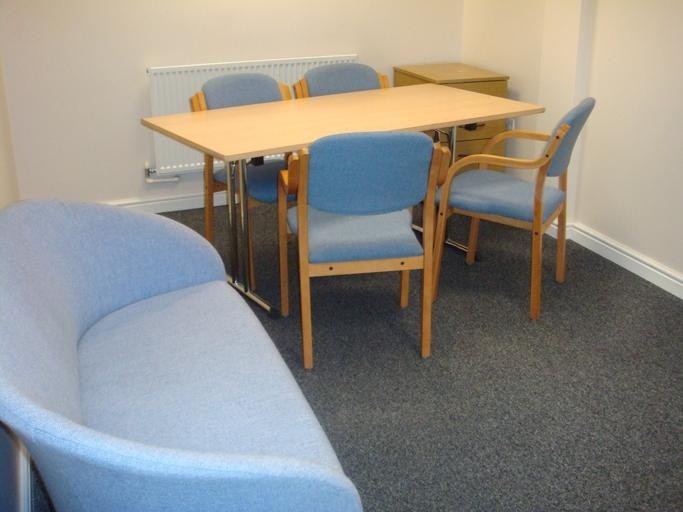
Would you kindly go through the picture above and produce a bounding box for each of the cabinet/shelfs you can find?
[394,62,510,173]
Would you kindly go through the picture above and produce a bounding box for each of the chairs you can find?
[431,98,595,321]
[294,62,390,98]
[190,74,292,245]
[279,130,453,370]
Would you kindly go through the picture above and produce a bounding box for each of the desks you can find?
[142,82,545,319]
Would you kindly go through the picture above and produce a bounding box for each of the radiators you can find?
[145,53,360,186]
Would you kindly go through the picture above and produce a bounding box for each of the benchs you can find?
[0,199,365,511]
